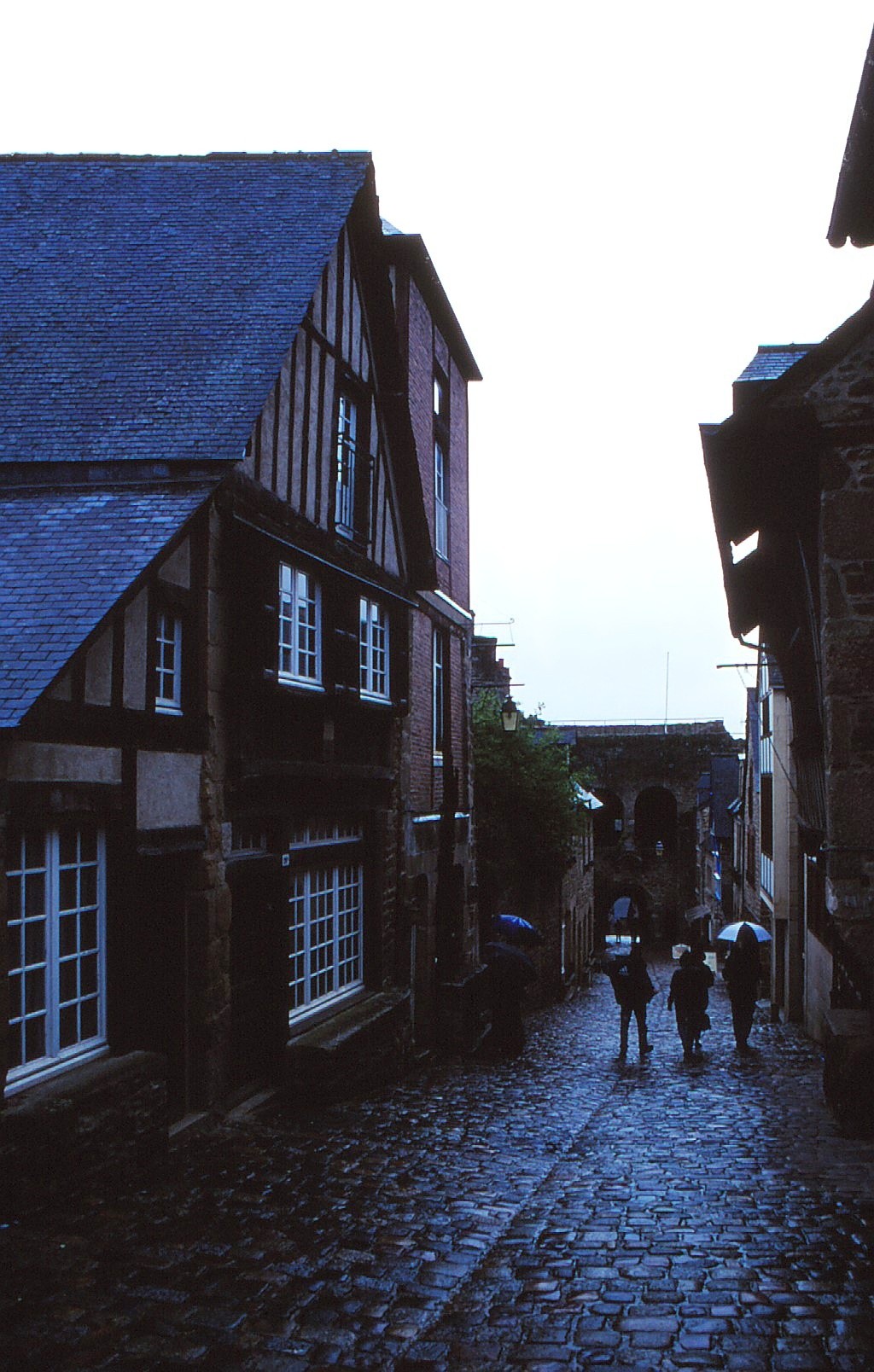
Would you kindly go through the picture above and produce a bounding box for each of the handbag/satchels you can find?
[700,1014,711,1031]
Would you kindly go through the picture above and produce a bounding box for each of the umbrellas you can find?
[490,914,548,947]
[717,921,773,945]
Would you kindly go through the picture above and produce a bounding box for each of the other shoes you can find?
[640,1045,654,1054]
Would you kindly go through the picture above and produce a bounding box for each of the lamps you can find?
[471,683,526,730]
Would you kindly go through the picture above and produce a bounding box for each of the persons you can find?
[727,943,763,1048]
[692,949,714,1048]
[619,942,654,1059]
[492,938,525,1057]
[608,887,639,941]
[667,950,703,1059]
